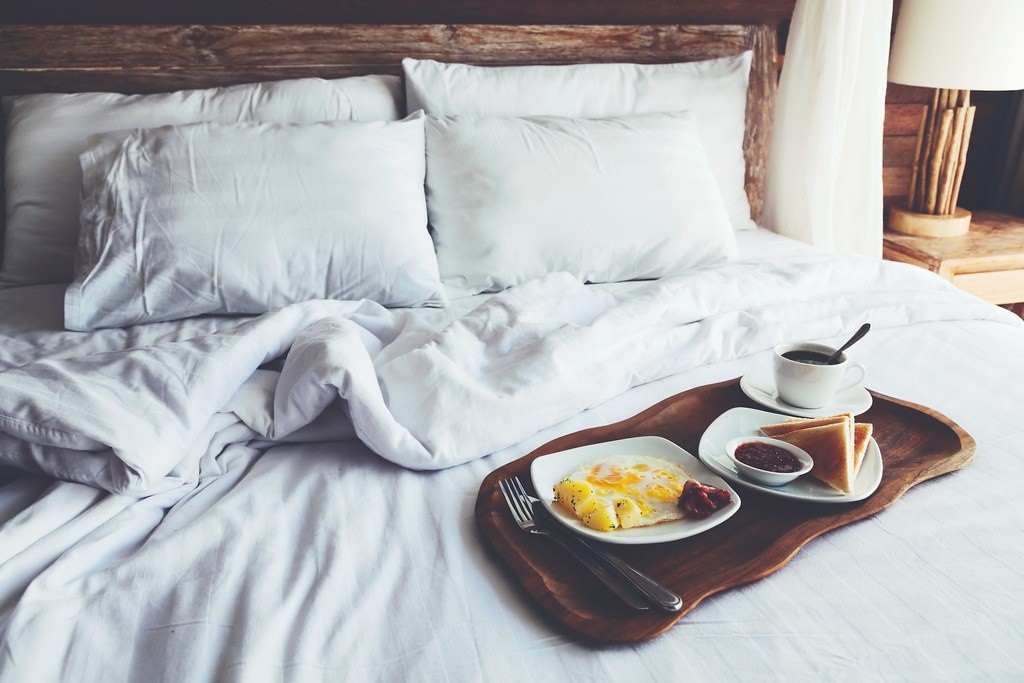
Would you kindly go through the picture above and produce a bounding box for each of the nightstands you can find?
[882,212,1024,310]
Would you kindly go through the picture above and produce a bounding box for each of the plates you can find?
[699,407,883,503]
[530,436,741,544]
[740,370,873,419]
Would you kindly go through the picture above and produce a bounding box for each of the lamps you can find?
[889,0,1024,237]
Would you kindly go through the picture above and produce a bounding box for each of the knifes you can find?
[519,495,682,612]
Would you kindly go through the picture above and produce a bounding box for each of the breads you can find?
[758,412,874,495]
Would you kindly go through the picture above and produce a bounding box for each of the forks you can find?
[498,477,654,612]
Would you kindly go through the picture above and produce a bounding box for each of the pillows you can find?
[1,74,402,291]
[399,49,760,233]
[63,107,450,333]
[419,110,743,304]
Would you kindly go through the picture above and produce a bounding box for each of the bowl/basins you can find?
[726,437,814,487]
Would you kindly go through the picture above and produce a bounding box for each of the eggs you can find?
[570,455,693,526]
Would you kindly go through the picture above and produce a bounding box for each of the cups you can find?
[773,341,865,408]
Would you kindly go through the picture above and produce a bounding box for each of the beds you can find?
[0,22,1024,683]
